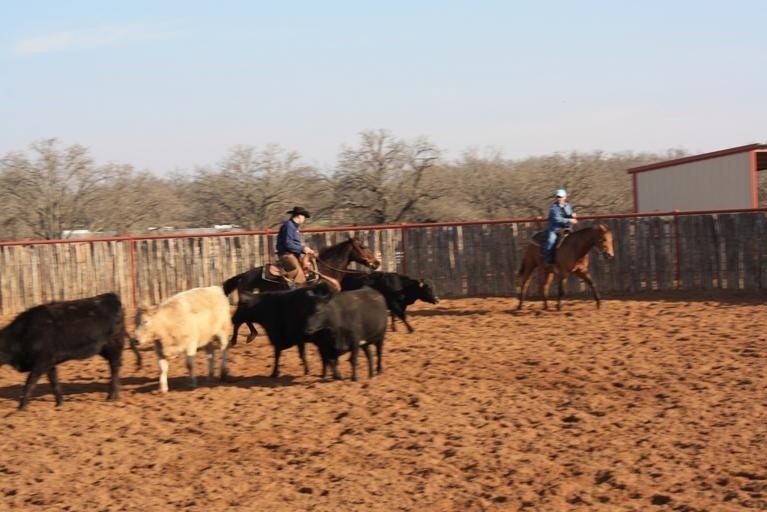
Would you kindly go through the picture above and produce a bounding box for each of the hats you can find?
[286,207,310,218]
[555,189,566,197]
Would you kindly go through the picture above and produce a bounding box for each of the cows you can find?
[0,292,143,412]
[230,283,335,378]
[132,284,232,395]
[304,286,387,381]
[341,268,439,335]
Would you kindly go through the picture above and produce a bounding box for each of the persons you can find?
[274,205,314,287]
[540,189,578,274]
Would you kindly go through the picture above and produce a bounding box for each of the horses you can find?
[223,234,379,346]
[516,223,613,313]
